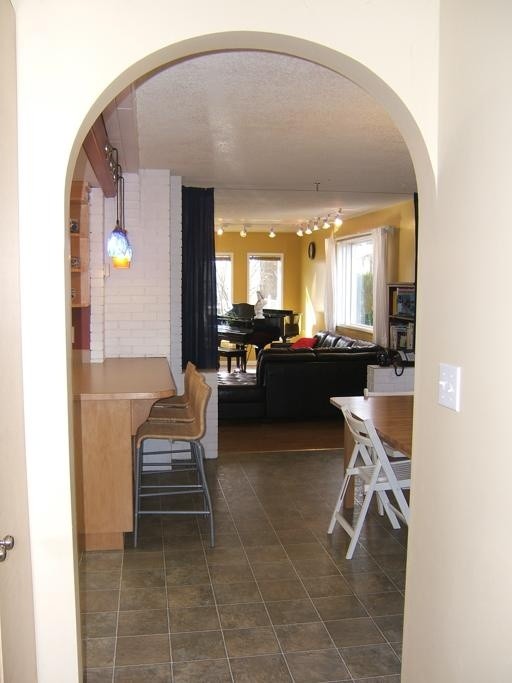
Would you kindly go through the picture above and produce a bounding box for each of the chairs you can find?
[326,404,412,561]
[138,362,203,486]
[137,371,209,519]
[135,382,216,548]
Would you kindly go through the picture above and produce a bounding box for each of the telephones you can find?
[392,349,415,376]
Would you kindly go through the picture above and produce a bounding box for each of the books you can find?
[389,284,420,318]
[387,323,417,349]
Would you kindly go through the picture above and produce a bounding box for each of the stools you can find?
[218,346,246,374]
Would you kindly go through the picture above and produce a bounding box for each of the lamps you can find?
[296,222,303,236]
[103,144,132,270]
[269,225,277,238]
[312,216,320,231]
[239,224,248,236]
[216,223,224,236]
[333,207,344,226]
[305,219,312,235]
[321,212,331,229]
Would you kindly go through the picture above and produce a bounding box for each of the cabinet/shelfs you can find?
[382,281,416,351]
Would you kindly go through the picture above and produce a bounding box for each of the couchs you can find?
[218,328,384,424]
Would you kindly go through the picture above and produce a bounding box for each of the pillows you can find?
[289,337,319,348]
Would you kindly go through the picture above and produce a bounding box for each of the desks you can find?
[329,395,413,510]
[79,356,177,552]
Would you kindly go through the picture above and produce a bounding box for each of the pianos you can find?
[217,303,303,369]
